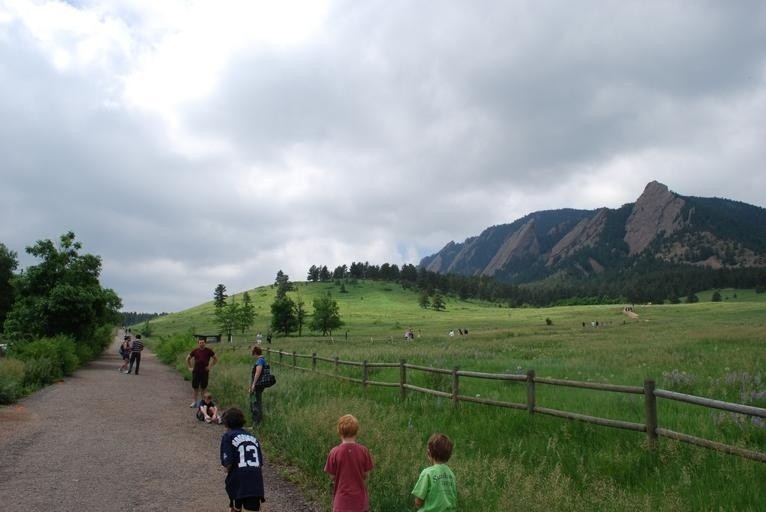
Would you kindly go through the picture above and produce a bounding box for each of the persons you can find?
[449,329,468,336]
[196,392,223,425]
[256,331,262,344]
[406,329,413,338]
[251,347,267,405]
[220,409,265,512]
[267,333,271,343]
[323,414,371,512]
[119,328,144,375]
[186,336,218,408]
[411,433,459,512]
[591,321,598,327]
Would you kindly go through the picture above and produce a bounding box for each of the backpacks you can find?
[119,345,124,354]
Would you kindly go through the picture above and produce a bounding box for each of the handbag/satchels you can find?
[252,365,270,385]
[256,374,276,389]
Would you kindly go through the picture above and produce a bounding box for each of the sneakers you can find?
[217,416,223,424]
[190,403,197,408]
[205,417,211,424]
